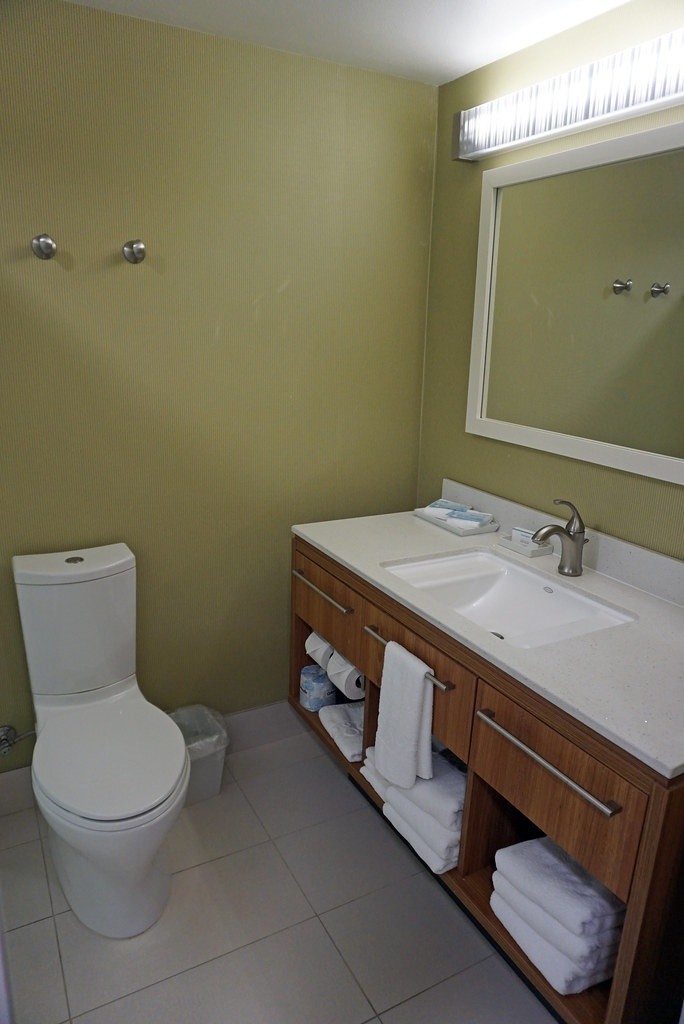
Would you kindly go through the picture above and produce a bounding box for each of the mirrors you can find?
[466,122,684,486]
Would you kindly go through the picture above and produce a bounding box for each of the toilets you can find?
[11,540,192,941]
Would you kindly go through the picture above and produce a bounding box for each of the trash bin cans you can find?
[168,705,229,808]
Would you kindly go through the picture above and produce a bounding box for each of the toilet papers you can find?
[304,631,366,700]
[298,664,338,714]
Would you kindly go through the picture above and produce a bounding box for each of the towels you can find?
[486,832,630,997]
[317,701,366,763]
[359,639,470,876]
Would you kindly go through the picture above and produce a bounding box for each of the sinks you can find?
[379,544,642,652]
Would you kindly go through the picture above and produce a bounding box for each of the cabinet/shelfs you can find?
[288,539,683,1024]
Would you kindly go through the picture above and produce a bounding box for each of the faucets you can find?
[530,498,589,578]
